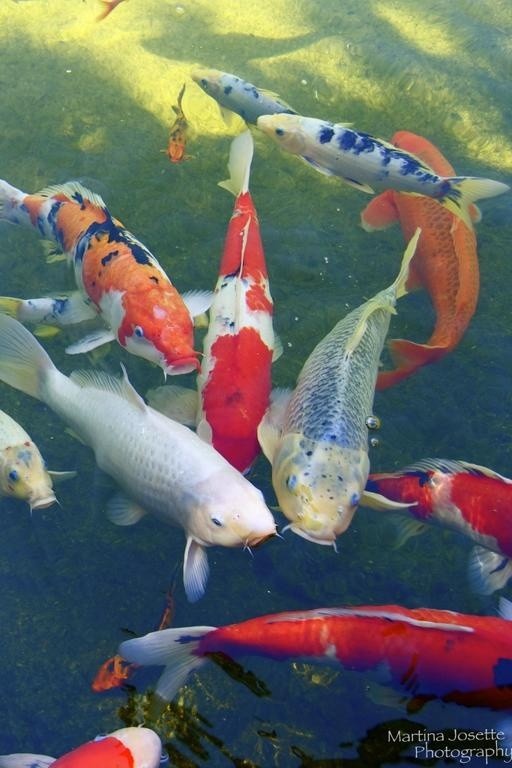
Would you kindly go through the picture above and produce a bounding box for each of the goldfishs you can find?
[2,64,509,596]
